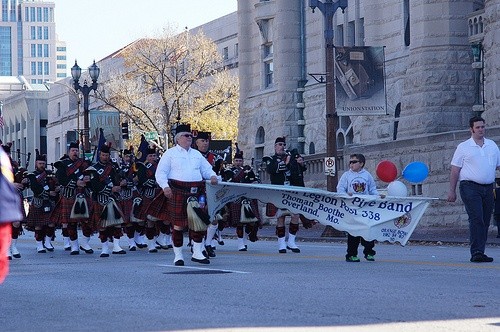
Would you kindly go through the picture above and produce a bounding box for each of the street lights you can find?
[70,59,101,161]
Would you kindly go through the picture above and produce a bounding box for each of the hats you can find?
[197,132,209,139]
[70,142,80,149]
[123,149,131,155]
[176,124,191,134]
[100,145,109,153]
[147,148,156,154]
[36,155,46,161]
[275,136,285,143]
[234,153,243,160]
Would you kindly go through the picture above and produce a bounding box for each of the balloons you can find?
[376,160,397,183]
[402,161,428,183]
[387,181,407,197]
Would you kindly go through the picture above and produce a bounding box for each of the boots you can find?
[191,242,211,264]
[238,238,246,251]
[205,227,216,257]
[278,236,286,253]
[217,231,224,245]
[288,233,300,252]
[7,227,173,261]
[173,246,185,266]
[210,240,216,250]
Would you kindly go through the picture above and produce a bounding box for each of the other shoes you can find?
[472,254,484,262]
[496,233,500,238]
[364,254,375,260]
[347,256,360,262]
[482,257,494,262]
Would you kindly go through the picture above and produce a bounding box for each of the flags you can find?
[0,115,4,128]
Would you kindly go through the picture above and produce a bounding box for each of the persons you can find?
[0,124,308,285]
[449,117,500,262]
[336,154,386,261]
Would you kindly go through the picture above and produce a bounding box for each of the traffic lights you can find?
[122,122,128,139]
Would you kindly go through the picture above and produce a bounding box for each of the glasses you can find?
[350,160,361,164]
[276,143,286,147]
[181,134,192,138]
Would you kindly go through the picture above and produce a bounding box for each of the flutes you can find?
[10,148,309,195]
